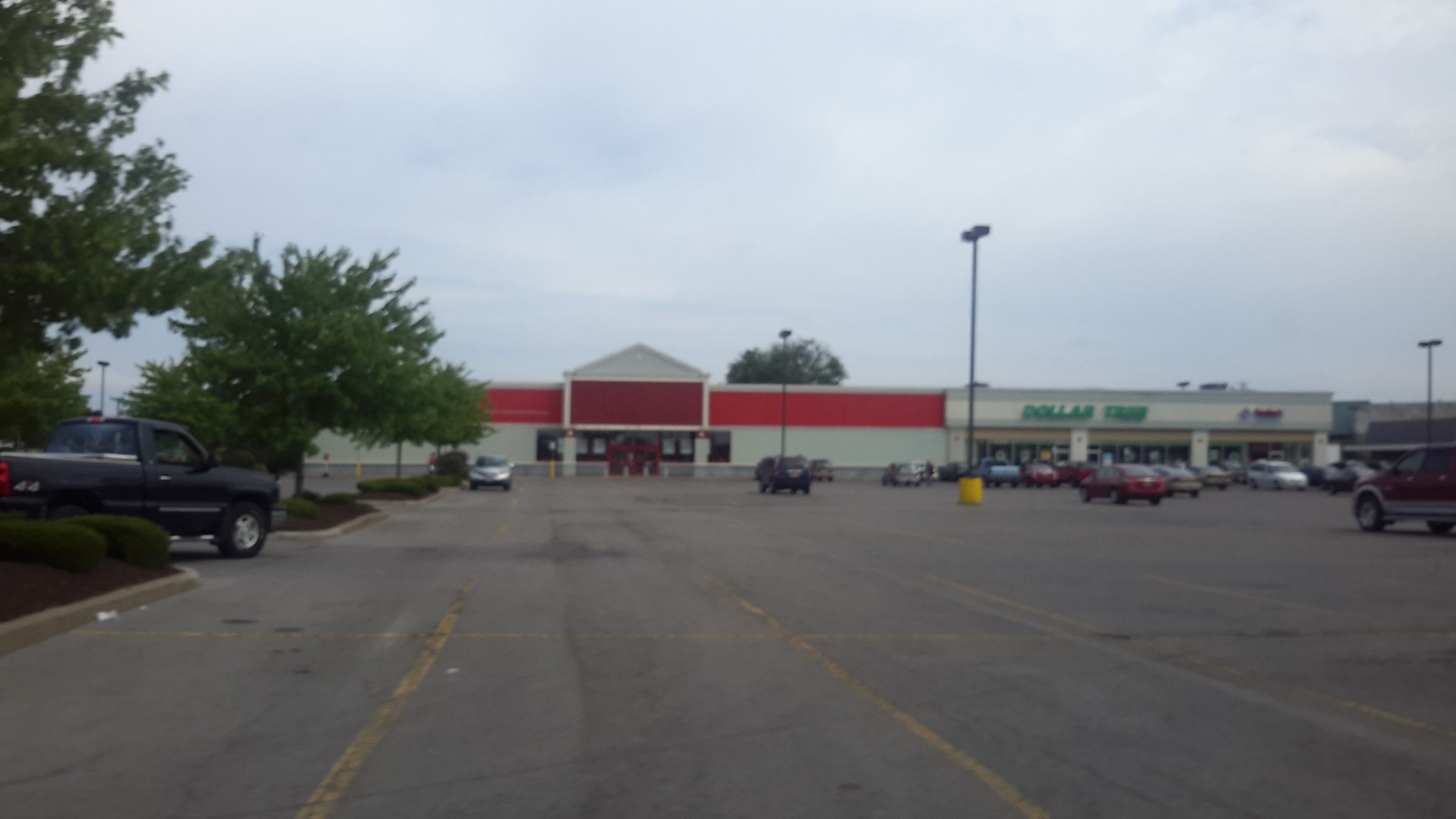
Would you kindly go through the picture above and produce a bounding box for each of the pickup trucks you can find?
[0,417,284,562]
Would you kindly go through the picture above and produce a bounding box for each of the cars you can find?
[468,454,516,491]
[426,451,475,480]
[809,458,835,482]
[1243,458,1310,492]
[1186,464,1230,490]
[917,456,1094,490]
[881,461,923,488]
[1289,455,1396,493]
[1150,463,1203,500]
[1210,460,1249,484]
[1076,459,1171,504]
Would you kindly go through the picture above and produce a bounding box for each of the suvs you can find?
[1349,442,1456,537]
[756,454,814,494]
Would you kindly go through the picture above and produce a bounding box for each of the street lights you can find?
[778,328,792,458]
[97,359,111,419]
[1417,337,1444,443]
[960,224,992,472]
